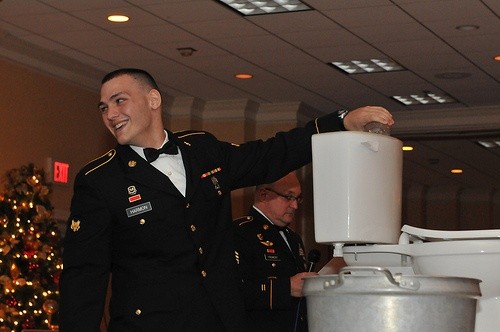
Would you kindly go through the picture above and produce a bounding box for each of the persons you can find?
[56,68,394,332]
[233,172,317,332]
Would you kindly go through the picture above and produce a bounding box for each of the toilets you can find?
[311,131,500,332]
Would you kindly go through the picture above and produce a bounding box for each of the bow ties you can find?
[143,139,179,163]
[273,225,291,238]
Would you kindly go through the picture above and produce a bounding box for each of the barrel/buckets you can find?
[301,266,483,332]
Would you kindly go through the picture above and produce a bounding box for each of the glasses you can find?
[265,187,304,205]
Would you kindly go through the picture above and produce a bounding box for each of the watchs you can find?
[338,109,348,131]
